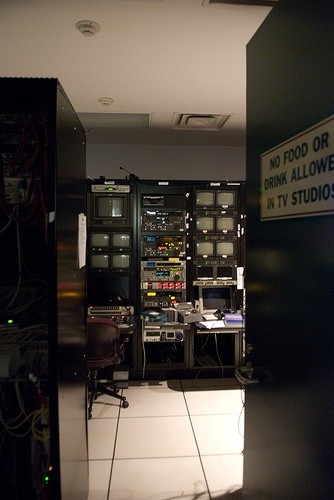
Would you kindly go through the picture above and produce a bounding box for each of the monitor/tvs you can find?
[94,194,127,221]
[195,189,240,279]
[198,286,236,314]
[90,231,132,274]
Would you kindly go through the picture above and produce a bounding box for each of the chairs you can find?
[86,319,129,420]
